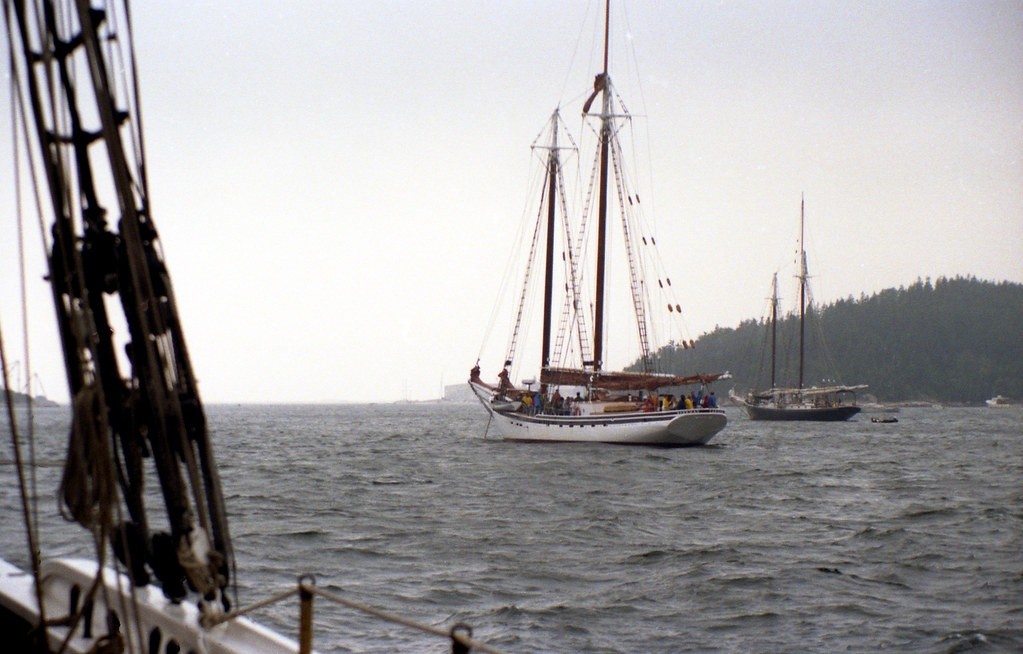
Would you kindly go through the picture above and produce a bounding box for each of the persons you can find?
[641,390,719,416]
[523,390,589,415]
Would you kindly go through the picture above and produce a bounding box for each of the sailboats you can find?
[466,0,733,449]
[727,190,869,422]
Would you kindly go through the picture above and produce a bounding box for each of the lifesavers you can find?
[645,400,655,412]
[831,401,838,408]
